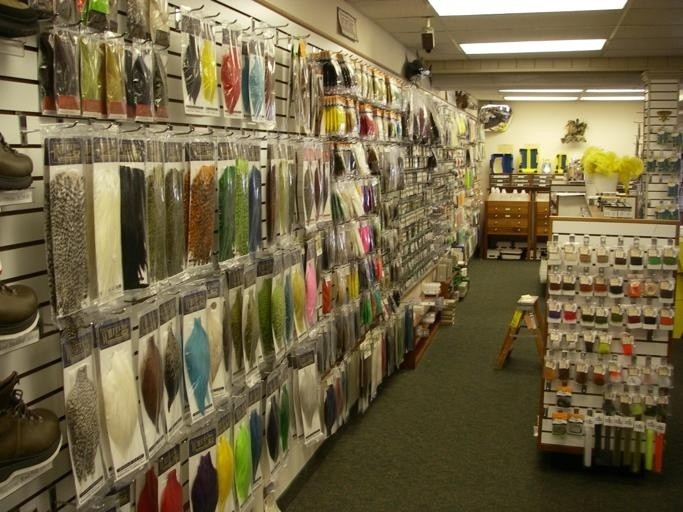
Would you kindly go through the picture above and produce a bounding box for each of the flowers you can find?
[580,146,646,194]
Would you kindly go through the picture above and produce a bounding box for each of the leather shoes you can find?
[0,278,40,336]
[0,0,41,39]
[0,130,35,192]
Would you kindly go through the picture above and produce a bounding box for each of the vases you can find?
[583,169,619,203]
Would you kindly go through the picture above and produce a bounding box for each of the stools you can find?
[495,295,546,370]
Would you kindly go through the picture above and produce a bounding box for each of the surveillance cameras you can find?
[421,29,435,53]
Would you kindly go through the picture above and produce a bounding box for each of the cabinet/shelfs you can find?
[483,186,532,260]
[532,188,551,259]
[0,37,55,501]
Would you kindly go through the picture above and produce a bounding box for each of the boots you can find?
[0,372,63,483]
